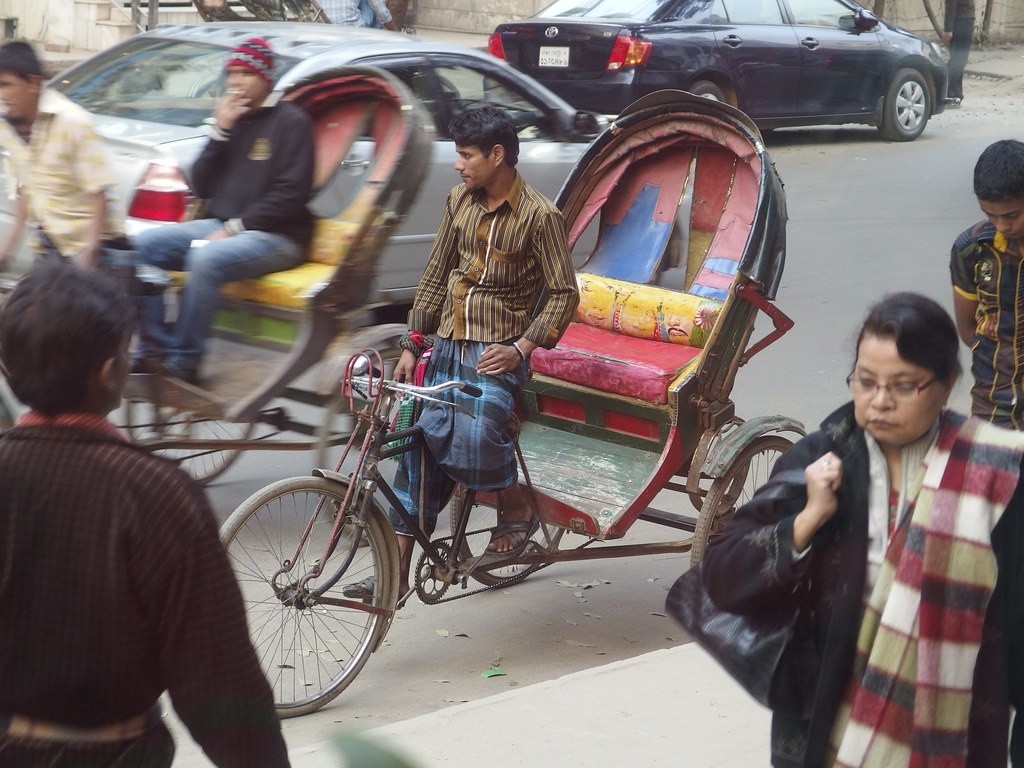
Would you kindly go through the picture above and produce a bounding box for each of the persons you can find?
[0,40,134,284]
[315,0,397,30]
[127,38,317,381]
[701,291,1024,768]
[0,260,292,767]
[949,140,1023,430]
[343,107,581,607]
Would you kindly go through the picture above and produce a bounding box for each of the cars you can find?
[31,20,630,363]
[484,2,963,143]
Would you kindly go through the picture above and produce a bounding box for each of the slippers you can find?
[484,516,539,558]
[343,576,406,608]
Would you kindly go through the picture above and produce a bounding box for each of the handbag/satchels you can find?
[665,470,814,709]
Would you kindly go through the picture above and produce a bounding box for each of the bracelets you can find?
[513,342,525,361]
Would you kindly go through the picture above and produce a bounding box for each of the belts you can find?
[7,715,145,740]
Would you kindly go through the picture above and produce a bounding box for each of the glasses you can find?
[846,366,942,398]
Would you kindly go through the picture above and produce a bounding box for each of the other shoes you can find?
[127,354,160,375]
[153,358,200,387]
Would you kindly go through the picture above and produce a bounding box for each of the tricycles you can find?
[104,65,436,482]
[206,86,807,720]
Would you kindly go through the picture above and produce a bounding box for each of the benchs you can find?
[169,220,357,353]
[529,272,724,454]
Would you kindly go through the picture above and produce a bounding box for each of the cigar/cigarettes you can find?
[225,90,239,94]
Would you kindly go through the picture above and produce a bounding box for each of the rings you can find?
[823,458,831,465]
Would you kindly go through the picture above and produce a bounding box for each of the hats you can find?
[226,37,274,92]
